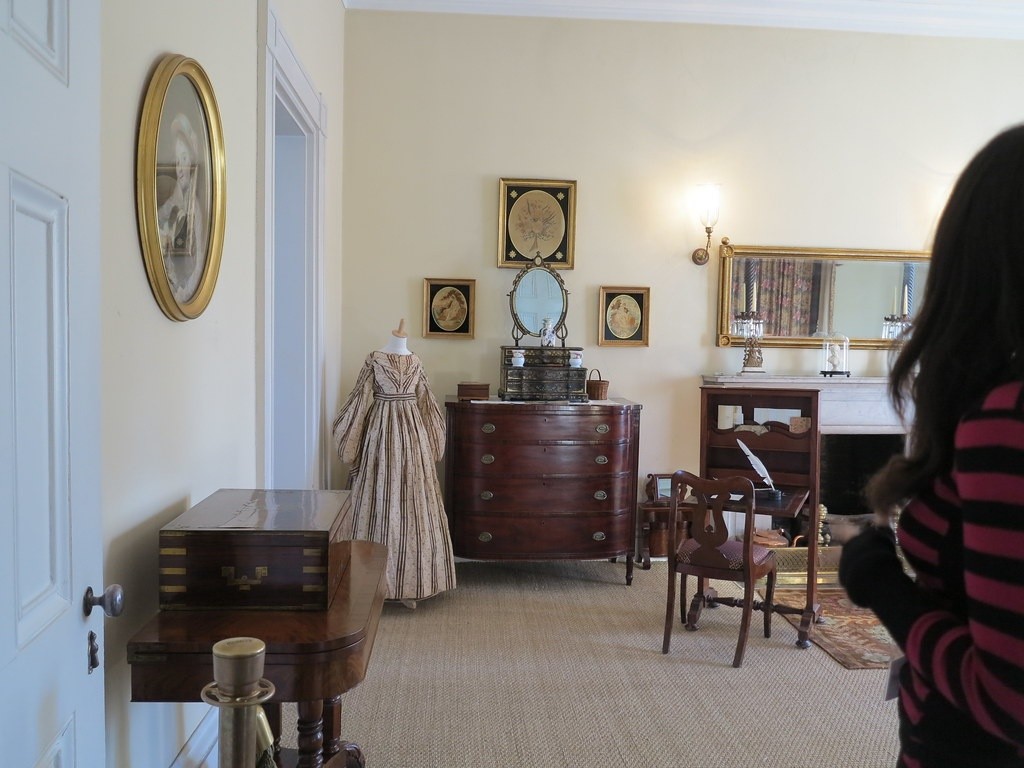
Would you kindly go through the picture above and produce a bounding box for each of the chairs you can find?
[661,470,778,668]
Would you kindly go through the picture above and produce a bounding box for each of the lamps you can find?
[691,199,720,265]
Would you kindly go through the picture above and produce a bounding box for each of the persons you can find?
[332,319,456,608]
[840,124,1024,768]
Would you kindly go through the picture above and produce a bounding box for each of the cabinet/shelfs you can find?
[443,394,645,586]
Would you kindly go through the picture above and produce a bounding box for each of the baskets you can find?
[586,368,610,399]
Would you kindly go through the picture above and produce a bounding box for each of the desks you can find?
[123,541,391,768]
[682,384,828,650]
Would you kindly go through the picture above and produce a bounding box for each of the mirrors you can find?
[716,237,932,350]
[507,251,569,348]
[653,473,672,502]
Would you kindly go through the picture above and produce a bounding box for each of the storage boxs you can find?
[157,487,358,613]
[497,346,589,402]
[457,381,490,401]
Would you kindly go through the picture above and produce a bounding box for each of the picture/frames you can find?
[496,175,577,271]
[135,49,229,321]
[422,277,475,340]
[599,286,650,348]
